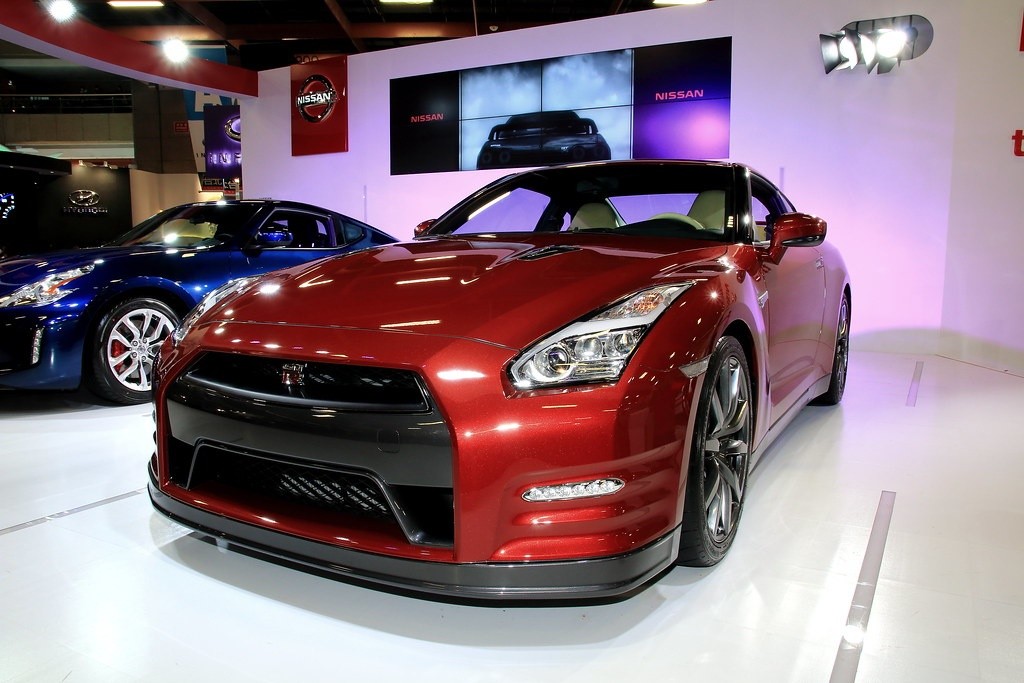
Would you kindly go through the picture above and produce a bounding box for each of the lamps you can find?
[819,14,934,74]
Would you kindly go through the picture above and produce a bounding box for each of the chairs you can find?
[568,203,616,232]
[687,190,725,229]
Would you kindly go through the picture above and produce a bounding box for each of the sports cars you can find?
[149,159,851,599]
[475,111,611,169]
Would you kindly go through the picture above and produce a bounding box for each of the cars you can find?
[0,196,402,406]
